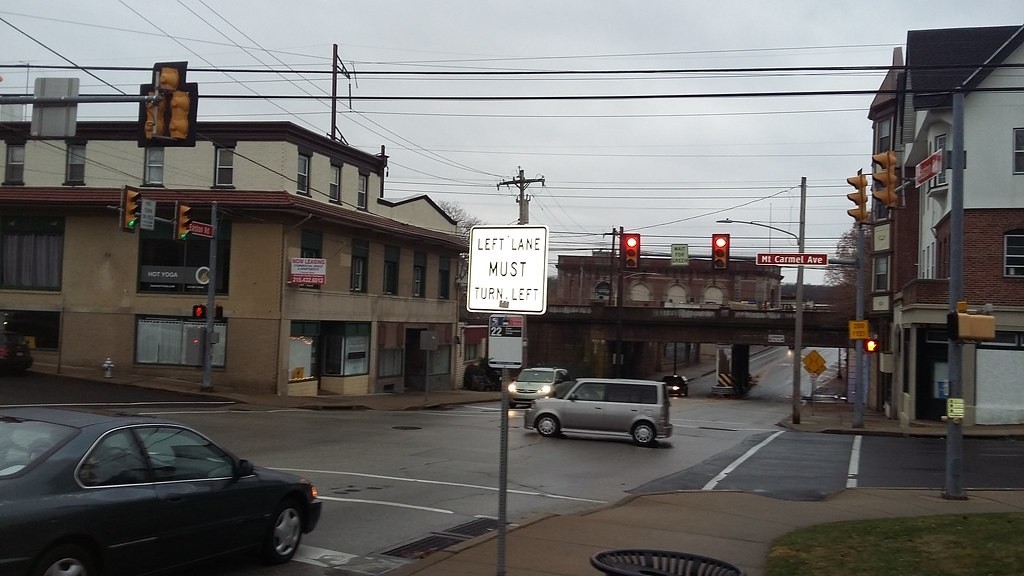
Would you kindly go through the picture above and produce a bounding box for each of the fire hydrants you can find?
[103,357,114,378]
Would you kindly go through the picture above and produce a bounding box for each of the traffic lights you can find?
[193,304,206,318]
[622,233,640,271]
[872,151,898,209]
[846,168,869,223]
[711,234,730,272]
[864,337,879,353]
[119,185,141,233]
[173,199,194,242]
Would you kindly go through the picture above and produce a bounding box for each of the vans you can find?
[523,378,674,447]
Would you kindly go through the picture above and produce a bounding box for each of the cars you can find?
[0,405,322,576]
[662,375,689,397]
[508,366,574,409]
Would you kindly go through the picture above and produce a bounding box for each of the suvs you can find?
[0,331,33,375]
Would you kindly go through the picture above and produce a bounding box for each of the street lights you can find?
[716,218,806,425]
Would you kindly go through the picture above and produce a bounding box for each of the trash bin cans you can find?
[591,549,747,576]
[490,371,502,390]
